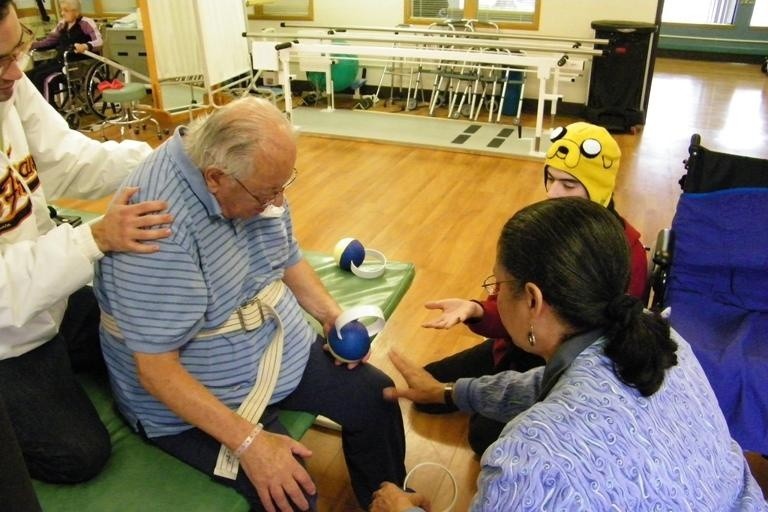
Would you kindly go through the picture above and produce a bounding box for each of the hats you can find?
[544,121,621,208]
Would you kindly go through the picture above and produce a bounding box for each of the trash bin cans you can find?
[583,20,658,136]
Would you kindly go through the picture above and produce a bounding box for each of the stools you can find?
[101,82,163,141]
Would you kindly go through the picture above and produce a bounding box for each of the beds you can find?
[0,203,416,512]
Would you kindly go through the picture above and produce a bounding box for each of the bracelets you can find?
[232,422,265,460]
[442,381,456,411]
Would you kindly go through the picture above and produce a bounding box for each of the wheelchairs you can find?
[646,134,767,460]
[27,18,128,129]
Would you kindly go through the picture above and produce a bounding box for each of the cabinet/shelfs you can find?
[106,28,153,94]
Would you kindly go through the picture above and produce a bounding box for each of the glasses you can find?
[0,23,34,78]
[235,168,298,206]
[482,275,515,292]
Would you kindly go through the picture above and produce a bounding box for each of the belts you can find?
[100,282,286,481]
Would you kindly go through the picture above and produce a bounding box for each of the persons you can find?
[93,95,419,512]
[366,195,768,512]
[0,1,173,511]
[412,121,647,456]
[32,0,104,105]
